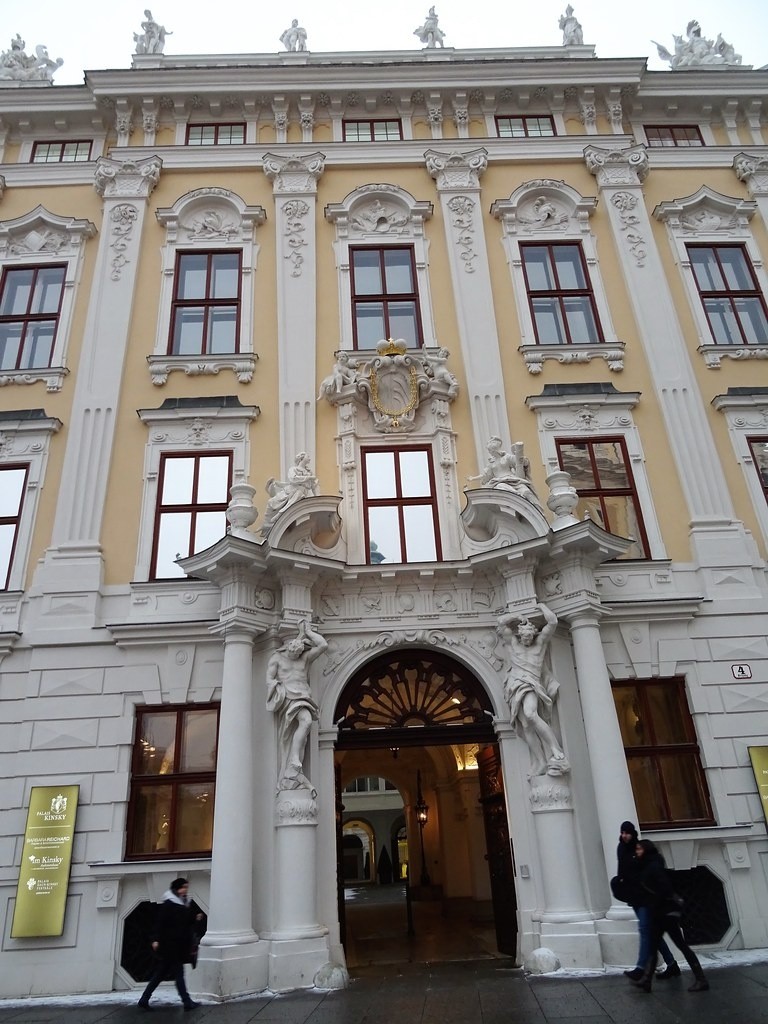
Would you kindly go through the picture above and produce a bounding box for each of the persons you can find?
[316,351,354,401]
[138,878,202,1012]
[267,619,328,769]
[630,838,710,993]
[422,343,458,387]
[254,452,320,534]
[497,603,571,781]
[617,821,681,983]
[464,441,545,516]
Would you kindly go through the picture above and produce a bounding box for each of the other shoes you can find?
[689,975,709,992]
[658,963,681,979]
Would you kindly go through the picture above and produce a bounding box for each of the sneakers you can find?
[623,968,644,980]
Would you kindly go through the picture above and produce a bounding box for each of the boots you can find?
[632,963,657,993]
[179,992,202,1011]
[137,995,151,1011]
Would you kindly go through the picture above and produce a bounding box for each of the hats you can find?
[170,878,189,889]
[620,821,635,833]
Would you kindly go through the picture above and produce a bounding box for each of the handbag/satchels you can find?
[655,892,687,932]
